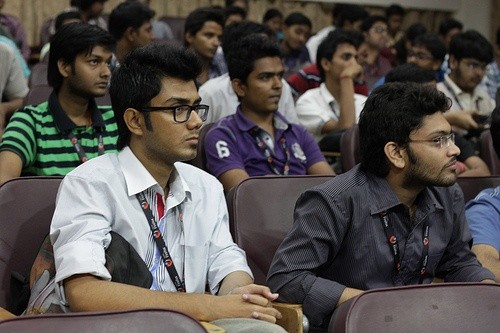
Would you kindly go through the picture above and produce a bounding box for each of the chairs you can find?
[1,15,500,333]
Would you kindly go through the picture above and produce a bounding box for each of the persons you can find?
[465,105,500,283]
[0,0,500,186]
[265,81,495,333]
[49,44,285,333]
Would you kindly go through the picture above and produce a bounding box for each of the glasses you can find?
[138,103,211,124]
[406,132,456,148]
[407,50,436,60]
[371,26,390,33]
[461,61,485,69]
